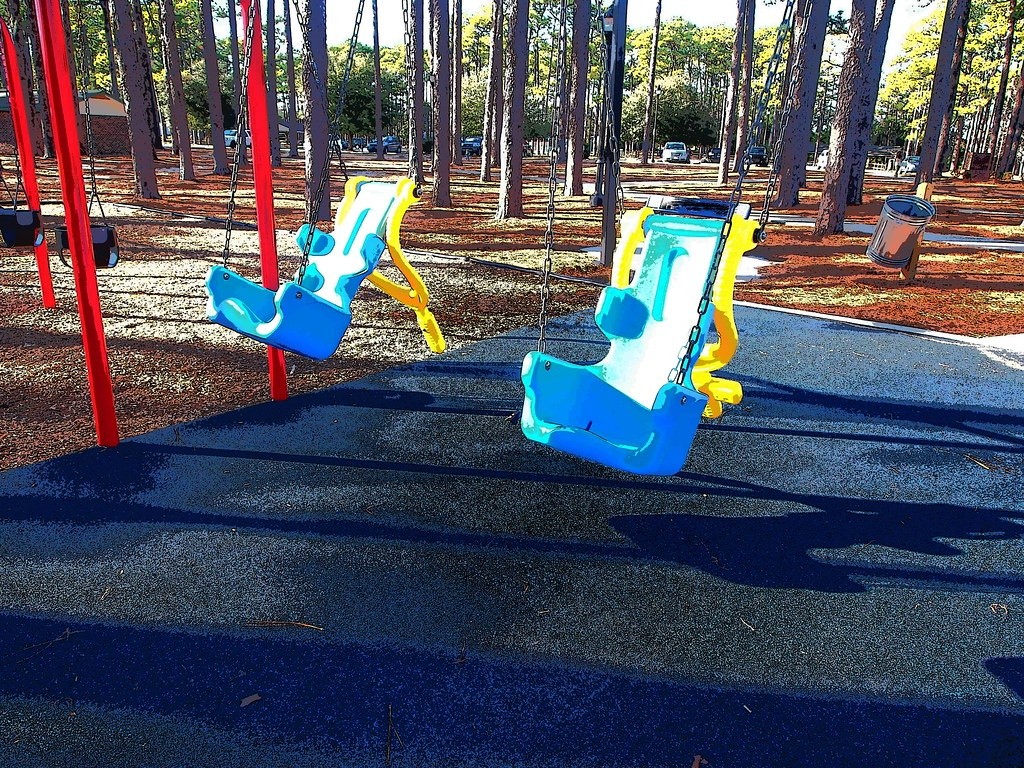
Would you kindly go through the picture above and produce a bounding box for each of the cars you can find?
[328,133,366,150]
[744,146,769,167]
[367,136,403,154]
[224,130,251,148]
[707,148,721,163]
[660,142,691,164]
[898,155,921,173]
[817,148,829,169]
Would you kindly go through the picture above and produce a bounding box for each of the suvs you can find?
[460,137,483,157]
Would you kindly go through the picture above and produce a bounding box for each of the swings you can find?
[203,0,446,362]
[0,0,46,247]
[53,0,122,269]
[518,1,798,476]
